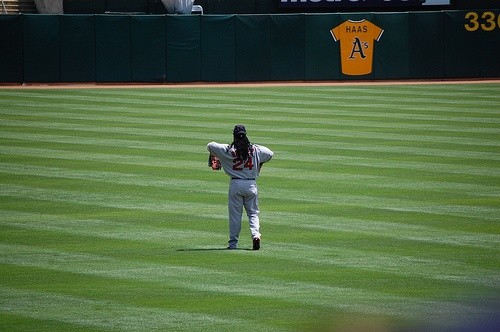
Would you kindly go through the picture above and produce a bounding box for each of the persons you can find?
[206,124,274,250]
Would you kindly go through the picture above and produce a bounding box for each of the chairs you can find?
[191,5,204,14]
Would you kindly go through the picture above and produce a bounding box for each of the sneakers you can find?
[253,237,260,250]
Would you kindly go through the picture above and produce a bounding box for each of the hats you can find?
[233,125,247,134]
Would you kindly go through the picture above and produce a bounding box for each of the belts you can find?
[230,177,256,180]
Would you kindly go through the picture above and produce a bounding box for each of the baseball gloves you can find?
[208,155,222,171]
